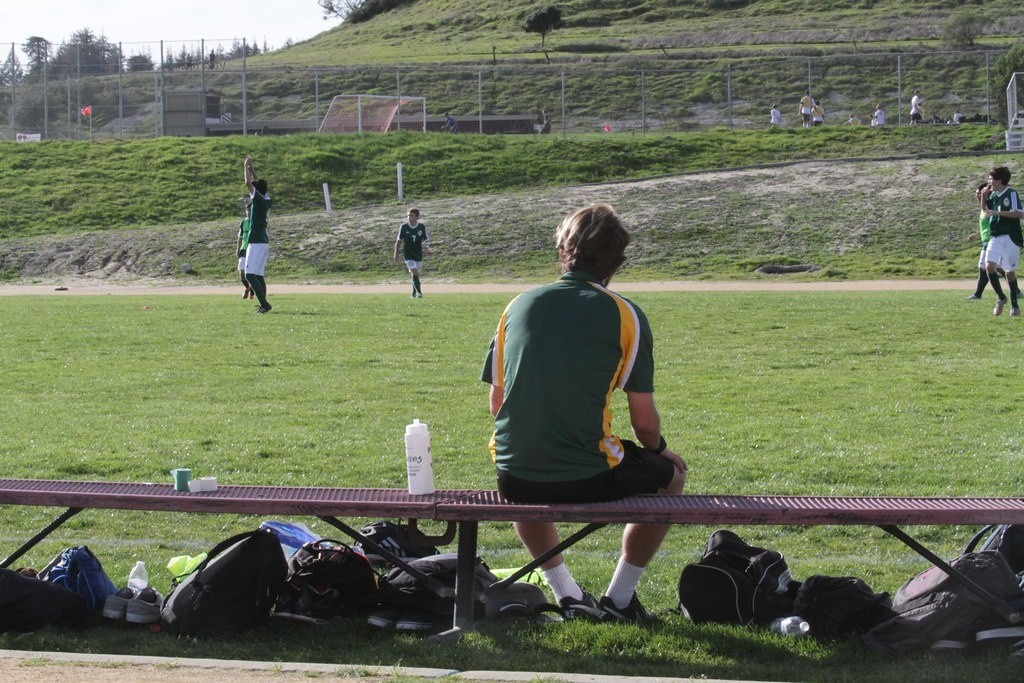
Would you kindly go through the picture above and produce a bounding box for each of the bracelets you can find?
[644,435,667,453]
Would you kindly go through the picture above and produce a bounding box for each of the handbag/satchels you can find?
[0,521,1024,659]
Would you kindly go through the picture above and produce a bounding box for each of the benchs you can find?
[0,478,1024,630]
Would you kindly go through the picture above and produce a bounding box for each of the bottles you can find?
[404,419,434,496]
[770,617,808,636]
[128,562,148,593]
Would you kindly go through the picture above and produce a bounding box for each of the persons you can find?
[186,54,192,70]
[771,105,780,127]
[965,183,1023,299]
[870,115,877,126]
[812,100,825,126]
[168,55,173,71]
[394,209,430,298]
[236,205,254,299]
[981,167,1024,316]
[244,157,272,313]
[910,91,922,124]
[441,113,459,134]
[209,50,215,69]
[480,203,688,620]
[875,105,884,126]
[943,110,961,123]
[798,90,818,127]
[848,114,862,126]
[540,110,552,133]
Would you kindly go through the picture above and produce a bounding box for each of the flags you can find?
[81,105,90,116]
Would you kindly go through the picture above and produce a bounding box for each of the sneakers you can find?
[964,295,981,300]
[411,293,416,299]
[293,585,353,627]
[1017,291,1023,298]
[595,590,659,621]
[1009,306,1021,316]
[366,603,434,631]
[993,297,1007,316]
[102,587,139,620]
[972,609,1024,645]
[255,303,272,314]
[249,290,254,299]
[417,293,423,298]
[273,580,304,620]
[558,590,599,620]
[929,622,970,652]
[126,586,165,624]
[243,287,250,299]
